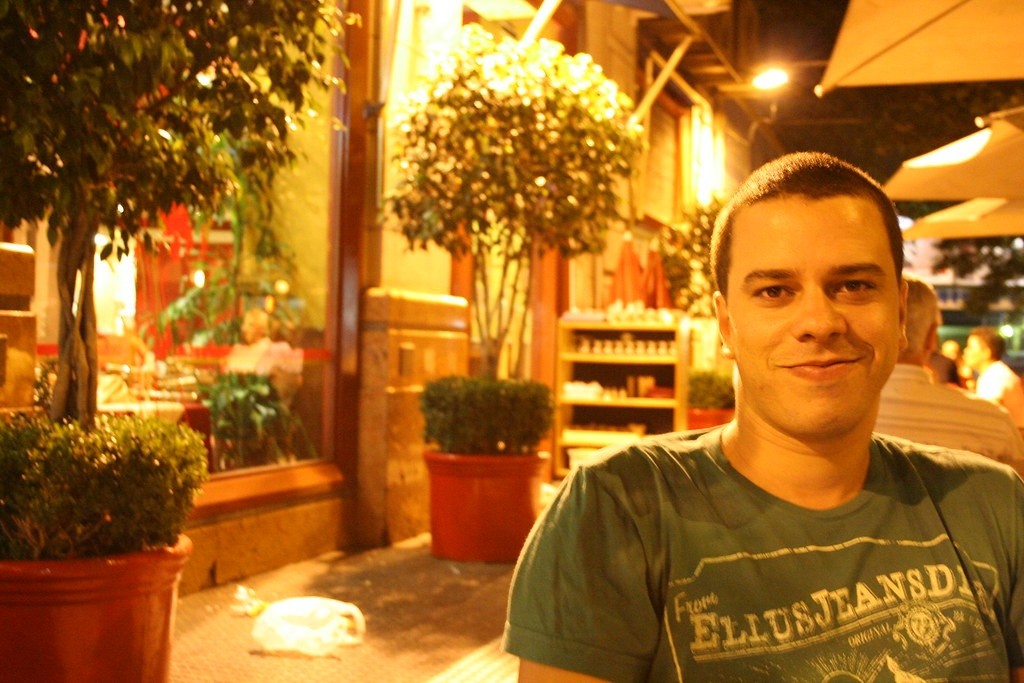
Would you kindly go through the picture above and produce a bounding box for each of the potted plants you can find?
[657,202,739,432]
[373,35,646,565]
[0,0,367,683]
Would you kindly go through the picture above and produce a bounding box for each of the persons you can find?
[501,150,1024,683]
[895,276,1024,439]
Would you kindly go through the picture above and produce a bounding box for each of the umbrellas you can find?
[815,0,1024,249]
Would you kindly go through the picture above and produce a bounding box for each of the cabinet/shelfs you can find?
[555,313,693,479]
